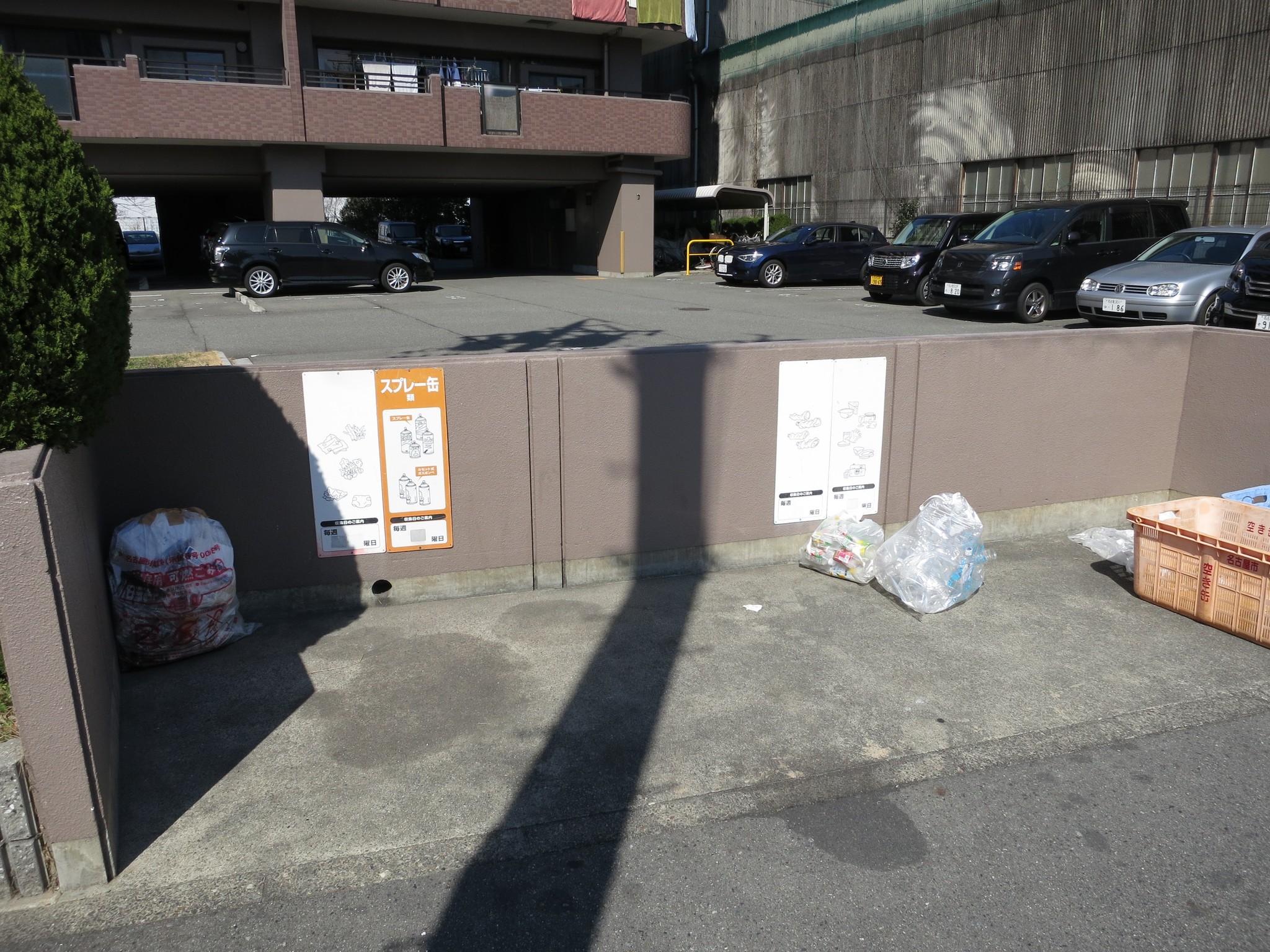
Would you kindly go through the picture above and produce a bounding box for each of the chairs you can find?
[1037,221,1058,242]
[826,229,834,242]
[854,230,863,240]
[1081,221,1100,241]
[1203,247,1228,262]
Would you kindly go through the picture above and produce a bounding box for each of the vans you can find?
[377,222,424,249]
[929,199,1191,323]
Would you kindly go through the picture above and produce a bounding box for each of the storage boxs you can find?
[1128,483,1270,650]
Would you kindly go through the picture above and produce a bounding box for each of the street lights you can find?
[138,217,146,231]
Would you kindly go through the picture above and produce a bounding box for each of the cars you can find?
[714,221,890,288]
[1076,225,1270,332]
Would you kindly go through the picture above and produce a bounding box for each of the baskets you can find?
[1221,485,1270,510]
[1127,496,1270,649]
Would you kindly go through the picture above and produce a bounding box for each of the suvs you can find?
[864,211,1004,307]
[425,223,471,257]
[210,222,435,298]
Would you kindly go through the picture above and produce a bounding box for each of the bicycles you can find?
[692,230,762,269]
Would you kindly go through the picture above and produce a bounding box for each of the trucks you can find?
[124,231,161,266]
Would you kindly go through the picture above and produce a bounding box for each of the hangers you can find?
[361,54,475,68]
